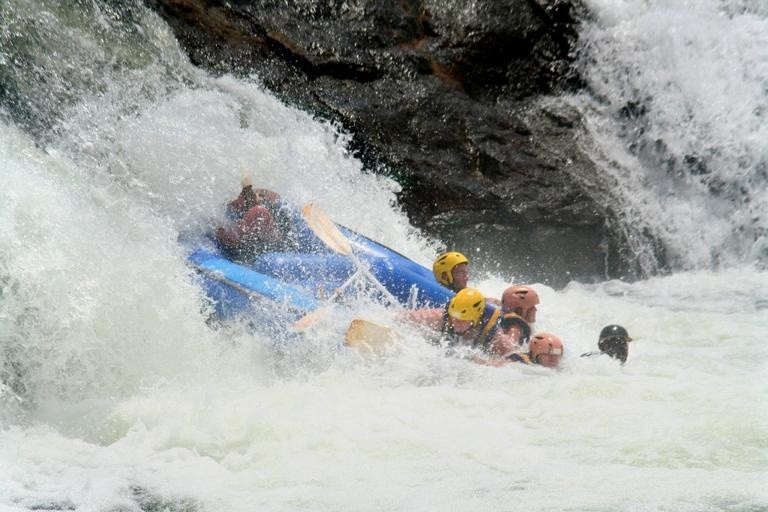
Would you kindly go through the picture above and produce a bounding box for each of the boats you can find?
[180,206,506,378]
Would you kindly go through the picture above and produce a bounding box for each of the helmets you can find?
[432,250,470,288]
[597,324,634,348]
[501,283,541,316]
[527,332,565,365]
[447,286,486,329]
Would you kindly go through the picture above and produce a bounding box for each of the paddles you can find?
[345,321,407,356]
[301,200,409,314]
[294,257,369,331]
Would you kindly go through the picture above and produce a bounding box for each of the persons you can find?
[398,288,529,369]
[432,252,468,294]
[523,333,564,369]
[579,324,632,366]
[500,284,540,347]
[216,178,285,262]
